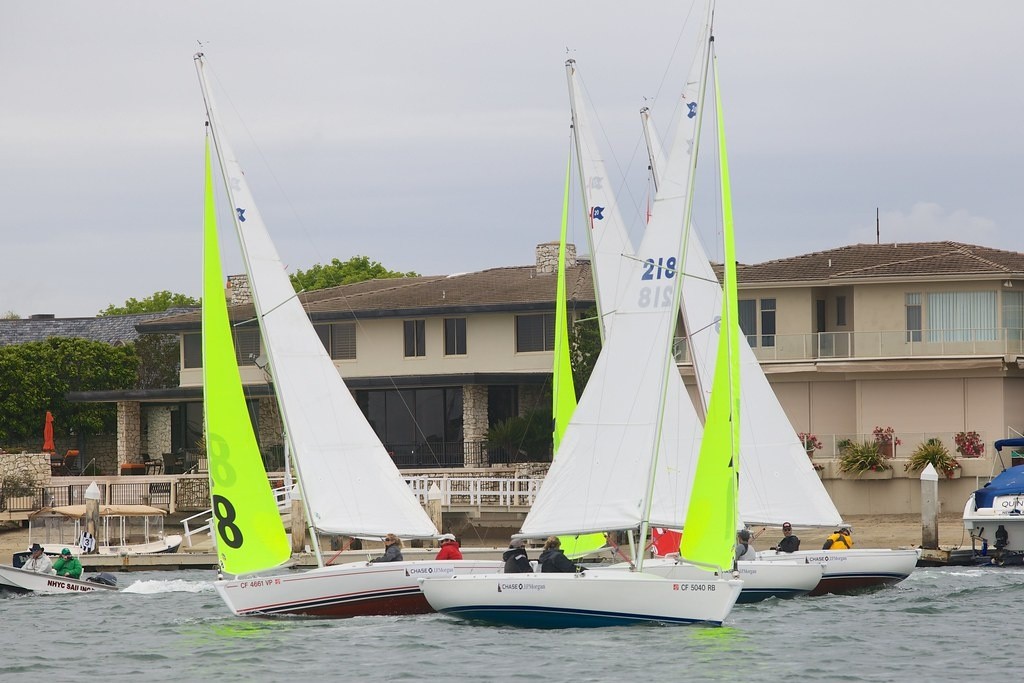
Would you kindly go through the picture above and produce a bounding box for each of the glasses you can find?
[385,538,392,541]
[784,529,790,531]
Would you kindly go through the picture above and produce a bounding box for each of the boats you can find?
[11,501,184,568]
[0,562,121,596]
[947,424,1024,569]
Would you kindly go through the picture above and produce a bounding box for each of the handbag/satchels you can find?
[86,573,117,586]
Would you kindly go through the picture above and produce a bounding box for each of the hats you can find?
[783,522,791,529]
[437,533,456,542]
[62,548,70,554]
[738,530,750,540]
[29,543,44,551]
[510,539,526,547]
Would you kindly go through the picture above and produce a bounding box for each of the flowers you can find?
[873,426,903,448]
[840,442,895,478]
[813,462,824,471]
[835,439,854,454]
[952,432,985,456]
[904,439,962,481]
[798,432,823,451]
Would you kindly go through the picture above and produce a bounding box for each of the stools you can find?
[120,464,146,476]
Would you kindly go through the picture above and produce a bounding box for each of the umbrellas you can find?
[43,412,55,452]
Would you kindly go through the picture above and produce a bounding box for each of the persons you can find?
[776,522,799,552]
[538,536,584,573]
[21,544,53,574]
[368,533,403,562]
[54,548,82,579]
[436,534,462,560]
[733,530,756,561]
[503,538,533,573]
[822,528,852,550]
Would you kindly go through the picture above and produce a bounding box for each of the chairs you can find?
[162,453,182,474]
[141,453,162,474]
[53,450,78,476]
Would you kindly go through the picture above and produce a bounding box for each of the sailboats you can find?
[632,94,924,599]
[543,45,827,604]
[188,37,542,622]
[395,1,745,625]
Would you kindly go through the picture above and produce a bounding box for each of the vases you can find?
[806,447,816,460]
[874,444,896,458]
[962,452,980,458]
[908,468,961,479]
[816,471,823,480]
[841,471,892,480]
[839,447,849,456]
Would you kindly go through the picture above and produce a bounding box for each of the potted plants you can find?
[3,476,35,509]
[196,438,208,470]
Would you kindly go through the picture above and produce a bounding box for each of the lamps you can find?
[1003,280,1012,287]
